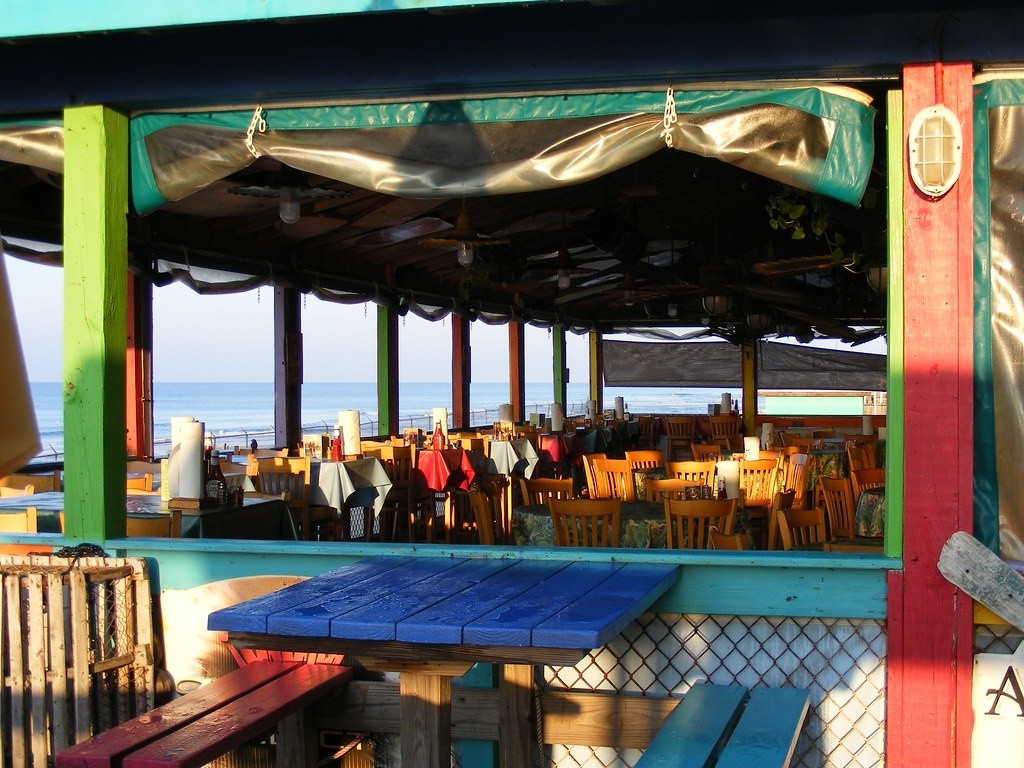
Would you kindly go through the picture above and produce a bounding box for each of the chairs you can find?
[235,415,887,551]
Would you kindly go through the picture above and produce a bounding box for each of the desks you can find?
[513,501,754,550]
[210,554,680,768]
[0,492,303,542]
[623,467,784,499]
[487,436,539,540]
[710,450,844,490]
[580,427,615,454]
[539,430,577,467]
[774,426,863,446]
[419,448,477,544]
[308,455,394,542]
[855,485,885,536]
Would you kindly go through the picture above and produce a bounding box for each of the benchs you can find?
[632,683,810,768]
[51,660,352,767]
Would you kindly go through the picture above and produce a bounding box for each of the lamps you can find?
[796,324,814,343]
[522,208,602,292]
[643,225,702,318]
[226,160,355,223]
[602,270,669,308]
[777,316,795,336]
[700,288,730,315]
[747,302,770,329]
[418,194,515,265]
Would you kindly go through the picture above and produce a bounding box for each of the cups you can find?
[227,489,244,504]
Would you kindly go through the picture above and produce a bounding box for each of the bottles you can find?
[326,447,332,459]
[216,481,226,504]
[331,424,343,462]
[433,422,444,450]
[205,450,226,502]
